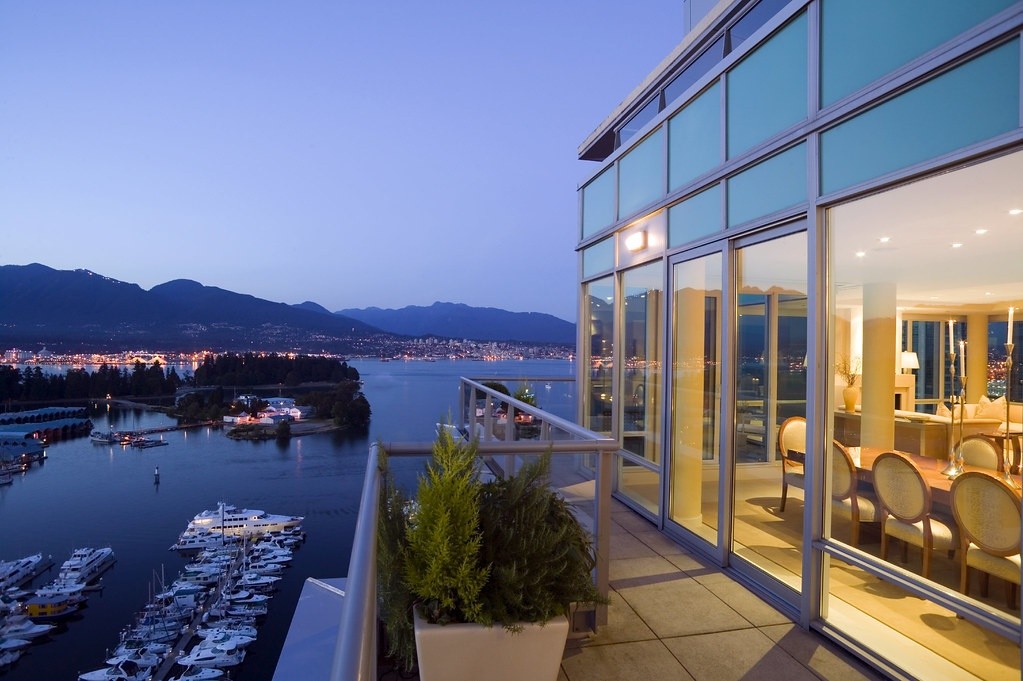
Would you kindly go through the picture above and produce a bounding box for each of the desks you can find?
[978,432,1021,475]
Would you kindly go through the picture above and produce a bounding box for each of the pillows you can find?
[936,402,968,420]
[973,394,1007,421]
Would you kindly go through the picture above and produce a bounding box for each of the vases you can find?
[843,385,859,414]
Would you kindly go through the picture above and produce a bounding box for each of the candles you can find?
[1007,307,1014,344]
[959,341,966,377]
[948,320,954,354]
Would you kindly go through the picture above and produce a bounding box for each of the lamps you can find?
[901,350,921,375]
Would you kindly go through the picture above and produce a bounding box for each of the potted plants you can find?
[374,405,613,681]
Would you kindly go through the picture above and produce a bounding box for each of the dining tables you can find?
[839,444,1022,509]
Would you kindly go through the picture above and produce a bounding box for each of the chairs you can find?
[870,450,991,601]
[954,434,1004,474]
[949,470,1023,619]
[777,414,806,512]
[831,439,909,564]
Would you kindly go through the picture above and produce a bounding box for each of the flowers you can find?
[832,353,861,386]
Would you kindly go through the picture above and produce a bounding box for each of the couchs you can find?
[952,402,1023,452]
[838,403,1002,449]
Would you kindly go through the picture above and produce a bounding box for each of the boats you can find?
[0,552,85,667]
[59,548,115,585]
[182,501,306,538]
[78,538,303,681]
[0,432,49,485]
[90,428,169,449]
